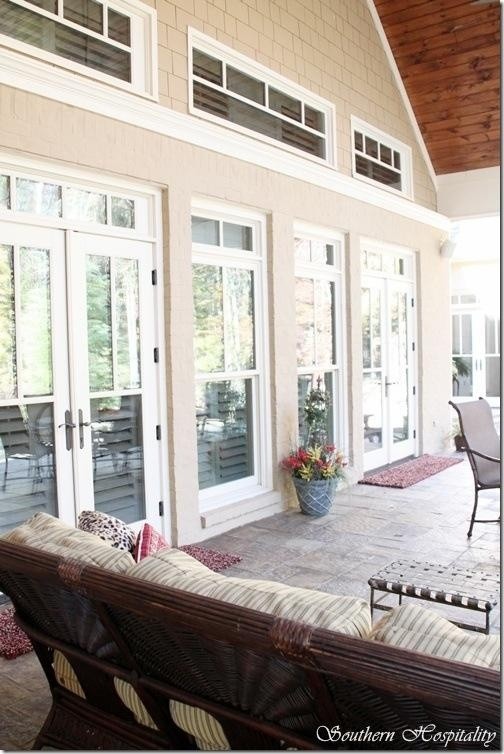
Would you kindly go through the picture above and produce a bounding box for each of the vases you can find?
[293,476,337,516]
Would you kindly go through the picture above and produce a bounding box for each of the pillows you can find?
[75,510,171,566]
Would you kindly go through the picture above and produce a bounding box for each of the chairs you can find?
[448,397,498,537]
[0,387,140,495]
[196,377,230,437]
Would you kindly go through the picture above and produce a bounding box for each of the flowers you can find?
[279,441,348,481]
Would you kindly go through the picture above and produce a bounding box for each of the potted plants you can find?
[445,423,466,452]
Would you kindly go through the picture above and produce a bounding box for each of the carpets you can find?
[0,543,241,660]
[356,453,464,488]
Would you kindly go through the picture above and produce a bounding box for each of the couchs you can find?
[0,512,499,751]
